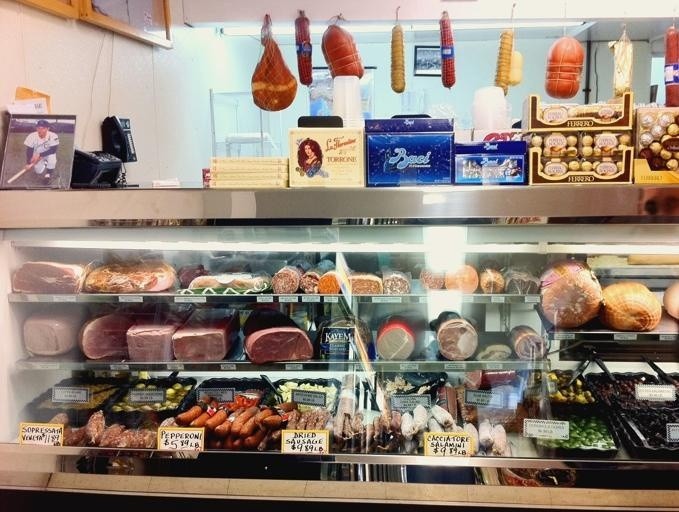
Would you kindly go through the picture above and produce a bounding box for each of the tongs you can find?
[565,349,620,388]
[399,372,447,400]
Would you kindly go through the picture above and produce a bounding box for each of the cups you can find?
[473,87,509,141]
[332,75,365,128]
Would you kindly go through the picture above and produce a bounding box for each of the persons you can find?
[24,120,60,185]
[297,139,323,177]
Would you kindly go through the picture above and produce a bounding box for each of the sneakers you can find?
[44,172,53,180]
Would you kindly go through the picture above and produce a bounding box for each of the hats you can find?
[34,119,53,129]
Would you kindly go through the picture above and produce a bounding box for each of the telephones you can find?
[101,116,139,165]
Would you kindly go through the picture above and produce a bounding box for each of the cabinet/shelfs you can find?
[1,185,679,512]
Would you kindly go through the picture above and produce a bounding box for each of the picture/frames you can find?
[308,65,379,124]
[0,112,76,190]
[21,1,81,20]
[81,0,175,51]
[414,44,442,76]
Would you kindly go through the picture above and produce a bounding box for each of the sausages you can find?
[178,394,293,450]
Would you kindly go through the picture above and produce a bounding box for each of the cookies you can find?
[271,258,412,293]
[444,264,480,290]
[418,260,444,289]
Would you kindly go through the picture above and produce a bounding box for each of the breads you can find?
[191,270,271,293]
[84,260,176,293]
